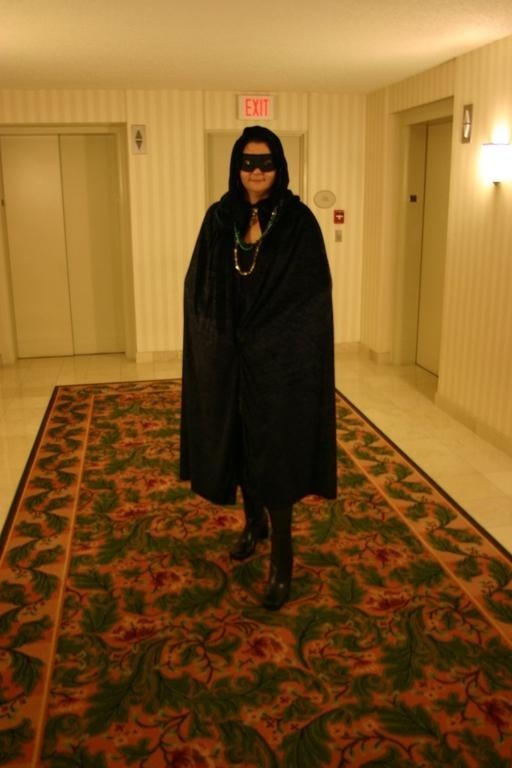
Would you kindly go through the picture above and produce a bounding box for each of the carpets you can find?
[1,376,512,768]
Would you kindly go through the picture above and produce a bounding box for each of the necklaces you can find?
[234,208,277,276]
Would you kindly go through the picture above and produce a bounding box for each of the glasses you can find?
[240,155,276,172]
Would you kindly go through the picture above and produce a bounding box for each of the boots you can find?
[230,513,290,611]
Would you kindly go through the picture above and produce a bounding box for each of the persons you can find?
[180,126,336,612]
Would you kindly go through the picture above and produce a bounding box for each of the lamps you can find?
[480,143,512,184]
